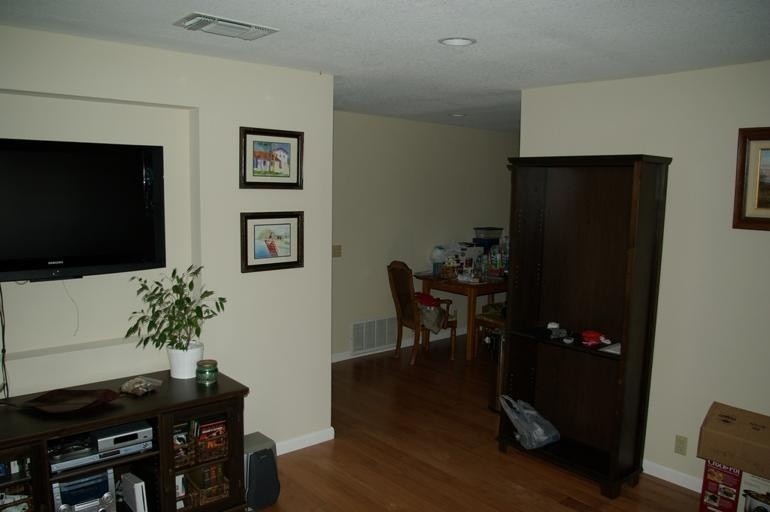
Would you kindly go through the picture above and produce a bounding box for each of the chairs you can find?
[386,260,458,367]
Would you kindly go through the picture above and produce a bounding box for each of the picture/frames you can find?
[729,127,769,232]
[238,126,306,273]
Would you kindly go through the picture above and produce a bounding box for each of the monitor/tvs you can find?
[0,138,166,282]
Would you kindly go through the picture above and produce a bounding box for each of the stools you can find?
[472,314,505,359]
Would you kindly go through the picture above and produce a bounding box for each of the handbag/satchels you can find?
[499,395,561,450]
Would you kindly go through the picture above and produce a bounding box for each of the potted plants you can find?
[124,265,227,380]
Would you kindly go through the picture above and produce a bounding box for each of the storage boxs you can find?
[693,402,769,511]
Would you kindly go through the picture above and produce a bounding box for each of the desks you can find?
[414,270,508,362]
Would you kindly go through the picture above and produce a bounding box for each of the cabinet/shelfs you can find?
[496,154,673,498]
[0,367,250,512]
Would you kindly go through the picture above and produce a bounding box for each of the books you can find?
[172,416,231,512]
